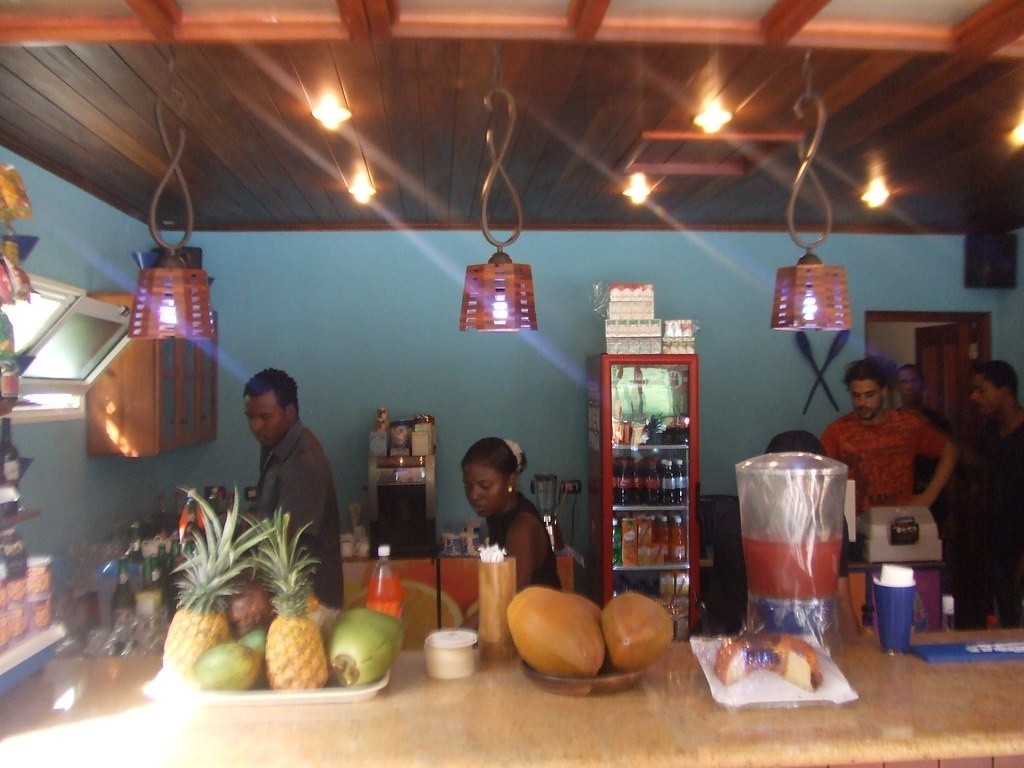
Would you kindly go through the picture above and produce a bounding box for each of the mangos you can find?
[236,629,266,664]
[191,644,259,690]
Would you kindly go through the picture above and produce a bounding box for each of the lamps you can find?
[773,51,851,332]
[459,88,538,333]
[128,86,214,341]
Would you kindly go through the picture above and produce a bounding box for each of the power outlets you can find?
[560,480,581,494]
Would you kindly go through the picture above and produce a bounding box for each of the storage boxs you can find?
[604,283,694,354]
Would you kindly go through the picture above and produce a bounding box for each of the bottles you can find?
[680,416,690,429]
[622,420,631,444]
[986,615,1000,630]
[1,417,22,516]
[614,458,687,504]
[613,515,686,564]
[110,524,192,625]
[368,545,404,620]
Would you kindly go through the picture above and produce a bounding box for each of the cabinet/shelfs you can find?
[86,292,216,457]
[0,164,65,693]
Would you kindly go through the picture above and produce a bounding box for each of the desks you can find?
[343,544,573,649]
[0,626,1024,768]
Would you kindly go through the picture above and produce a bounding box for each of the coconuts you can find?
[506,587,675,679]
[325,608,404,684]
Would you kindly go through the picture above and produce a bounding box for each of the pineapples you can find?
[161,484,332,691]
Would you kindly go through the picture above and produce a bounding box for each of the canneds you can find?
[1,554,54,656]
[389,420,414,449]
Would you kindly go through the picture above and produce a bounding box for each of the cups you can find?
[873,574,915,650]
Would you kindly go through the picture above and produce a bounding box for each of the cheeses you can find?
[714,632,824,693]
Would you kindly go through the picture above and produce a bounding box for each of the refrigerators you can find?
[584,354,699,632]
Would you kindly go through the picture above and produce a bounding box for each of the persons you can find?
[461,438,562,596]
[971,361,1024,628]
[238,368,343,609]
[707,430,863,638]
[893,364,970,538]
[819,358,957,516]
